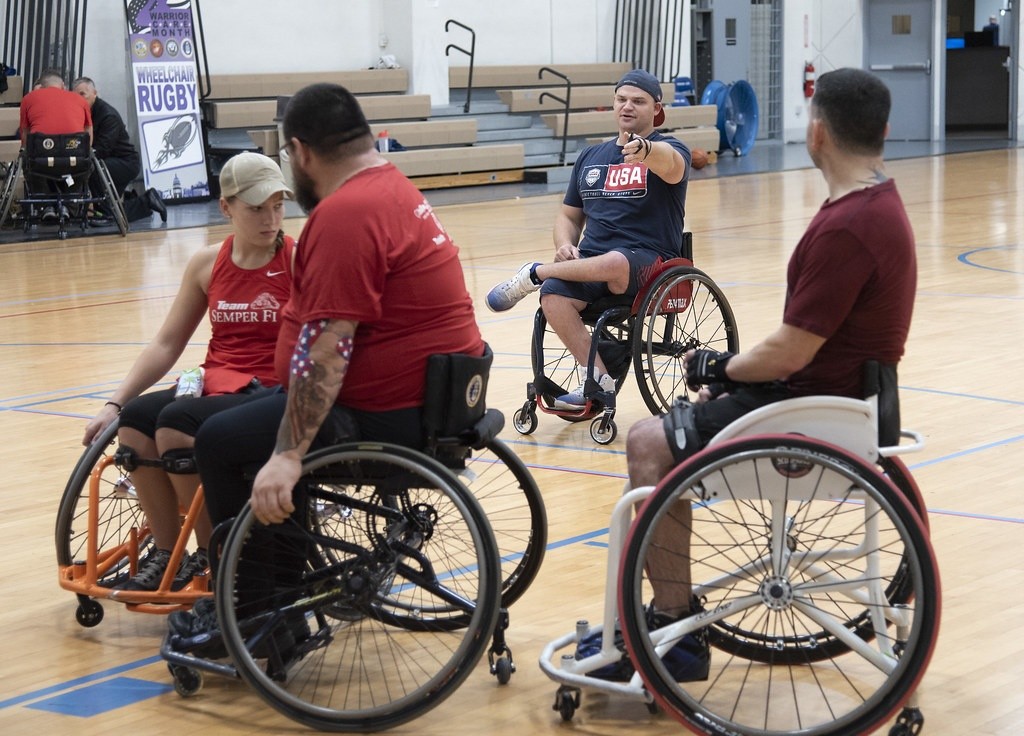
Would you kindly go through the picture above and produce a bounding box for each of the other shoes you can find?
[42,209,57,223]
[147,185,167,222]
[59,206,70,220]
[88,215,114,227]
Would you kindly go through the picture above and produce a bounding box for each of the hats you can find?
[218,146,295,206]
[613,68,666,127]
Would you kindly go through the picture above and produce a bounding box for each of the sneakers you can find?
[167,549,211,590]
[486,262,542,314]
[193,598,312,643]
[575,591,711,683]
[554,363,614,409]
[166,609,296,659]
[109,552,189,591]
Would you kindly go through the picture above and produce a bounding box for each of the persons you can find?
[82,151,298,607]
[485,73,692,410]
[577,66,917,683]
[16,69,167,222]
[166,80,484,658]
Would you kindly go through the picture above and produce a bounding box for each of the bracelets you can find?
[105,402,124,415]
[640,136,651,163]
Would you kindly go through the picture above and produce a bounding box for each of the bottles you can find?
[378,129,389,154]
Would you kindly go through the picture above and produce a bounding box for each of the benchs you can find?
[0,76,27,201]
[197,61,722,189]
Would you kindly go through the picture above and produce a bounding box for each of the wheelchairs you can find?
[539,358,942,736]
[512,233,740,445]
[54,342,548,732]
[0,131,129,241]
